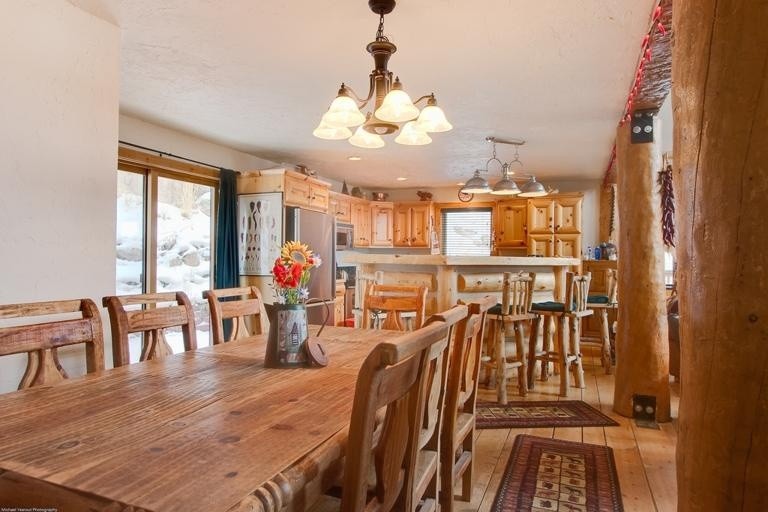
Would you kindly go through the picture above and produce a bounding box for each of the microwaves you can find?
[335,223,354,251]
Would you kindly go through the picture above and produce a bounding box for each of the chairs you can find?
[531,271,594,396]
[481,271,539,406]
[575,269,617,375]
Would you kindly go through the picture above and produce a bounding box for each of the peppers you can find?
[657,153,675,248]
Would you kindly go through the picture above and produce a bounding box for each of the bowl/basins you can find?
[372,191,389,202]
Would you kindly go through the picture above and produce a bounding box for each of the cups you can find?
[374,271,384,285]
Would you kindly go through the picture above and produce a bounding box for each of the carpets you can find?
[492,433,624,512]
[476,399,619,429]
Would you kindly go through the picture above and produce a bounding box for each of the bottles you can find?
[586,241,617,260]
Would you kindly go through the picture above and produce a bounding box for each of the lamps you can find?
[459,136,548,198]
[312,1,452,148]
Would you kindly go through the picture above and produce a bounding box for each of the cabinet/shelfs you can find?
[351,198,372,246]
[372,201,393,249]
[494,198,526,249]
[584,260,617,337]
[393,202,436,249]
[331,189,351,220]
[527,192,584,257]
[236,170,331,213]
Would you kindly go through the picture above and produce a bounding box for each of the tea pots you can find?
[261,297,333,369]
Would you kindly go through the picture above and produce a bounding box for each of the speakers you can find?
[631,118,653,144]
[633,395,656,422]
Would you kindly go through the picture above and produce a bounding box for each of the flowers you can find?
[270,242,324,303]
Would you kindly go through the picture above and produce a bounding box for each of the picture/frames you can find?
[235,191,283,277]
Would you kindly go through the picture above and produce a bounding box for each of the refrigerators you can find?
[285,205,336,325]
